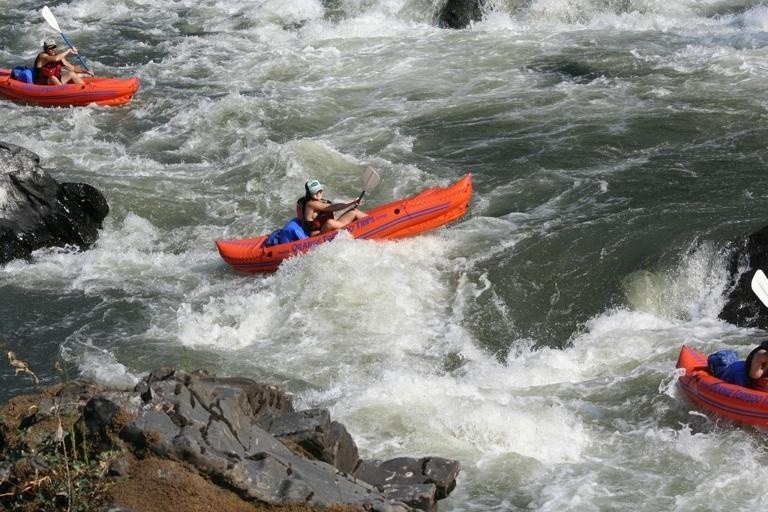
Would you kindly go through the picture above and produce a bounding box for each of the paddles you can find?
[751,269,768,307]
[354,165,380,207]
[42,5,89,72]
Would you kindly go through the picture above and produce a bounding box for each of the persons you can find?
[35,40,94,85]
[746,340,768,379]
[297,180,369,237]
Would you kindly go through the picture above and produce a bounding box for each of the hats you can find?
[304,178,326,196]
[43,38,57,49]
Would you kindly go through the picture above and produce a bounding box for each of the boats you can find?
[671,344,768,436]
[214,169,479,277]
[0,66,141,112]
